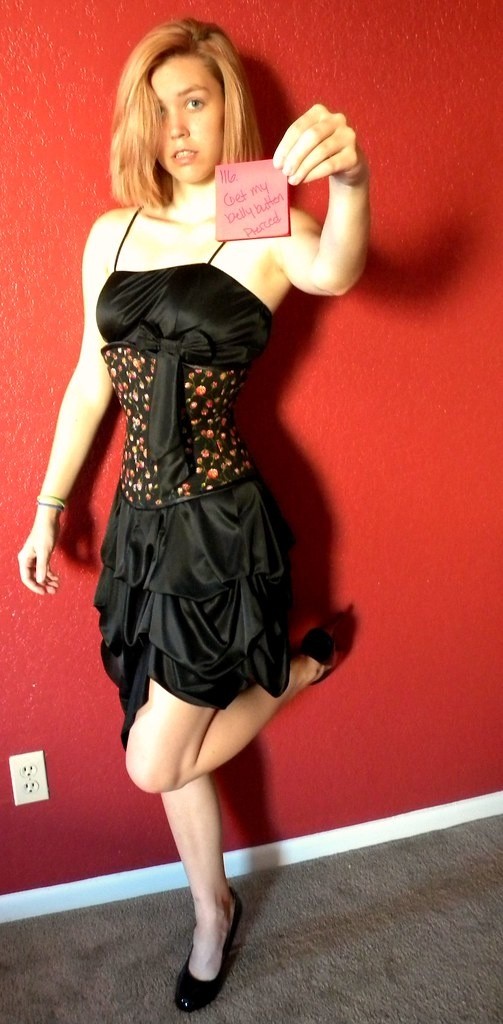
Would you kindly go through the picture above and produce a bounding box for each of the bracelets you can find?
[37,502,63,511]
[36,495,66,507]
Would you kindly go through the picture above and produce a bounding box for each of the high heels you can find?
[301,614,342,685]
[177,887,241,1012]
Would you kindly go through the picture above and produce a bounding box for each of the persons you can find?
[16,17,368,1014]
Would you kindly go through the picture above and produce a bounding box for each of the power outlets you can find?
[9,750,50,806]
